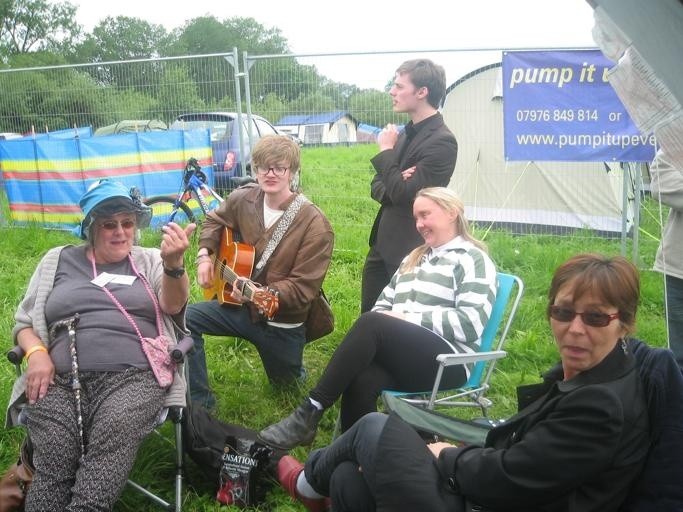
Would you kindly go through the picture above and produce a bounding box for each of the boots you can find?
[256,396,324,452]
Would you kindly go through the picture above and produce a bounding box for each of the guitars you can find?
[203,225,280,321]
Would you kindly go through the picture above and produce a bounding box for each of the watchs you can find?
[160,262,185,280]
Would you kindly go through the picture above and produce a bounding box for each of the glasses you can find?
[93,221,134,230]
[548,304,621,328]
[254,165,292,176]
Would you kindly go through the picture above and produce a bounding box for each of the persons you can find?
[360,59,458,314]
[12,177,197,512]
[184,133,335,409]
[277,255,651,511]
[651,145,683,378]
[257,186,500,456]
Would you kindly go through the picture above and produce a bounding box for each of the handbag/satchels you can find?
[302,286,336,344]
[0,435,36,512]
[140,332,176,389]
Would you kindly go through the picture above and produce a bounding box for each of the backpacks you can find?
[178,401,289,503]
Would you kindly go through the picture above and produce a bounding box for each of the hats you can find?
[77,178,153,239]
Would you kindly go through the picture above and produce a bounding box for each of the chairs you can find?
[4,294,196,511]
[327,270,527,445]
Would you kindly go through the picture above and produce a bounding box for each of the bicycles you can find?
[132,156,257,250]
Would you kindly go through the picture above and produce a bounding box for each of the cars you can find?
[168,110,302,191]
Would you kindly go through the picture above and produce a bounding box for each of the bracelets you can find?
[25,345,48,362]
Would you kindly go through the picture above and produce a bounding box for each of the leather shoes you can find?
[277,454,331,512]
